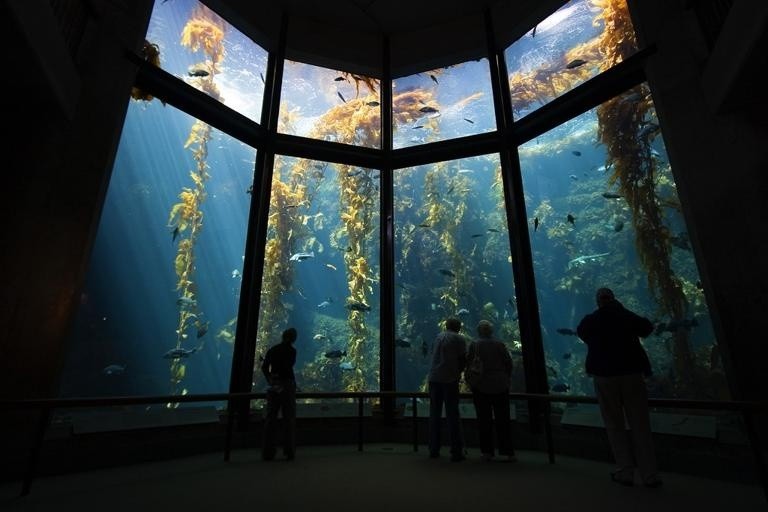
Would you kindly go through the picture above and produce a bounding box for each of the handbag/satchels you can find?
[465,355,482,386]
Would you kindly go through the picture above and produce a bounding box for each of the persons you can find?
[426,318,470,458]
[261,328,298,458]
[575,286,666,488]
[462,319,521,465]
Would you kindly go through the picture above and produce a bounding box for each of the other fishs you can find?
[258,150,694,409]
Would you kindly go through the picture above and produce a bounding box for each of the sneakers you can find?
[611,471,662,487]
[481,453,496,462]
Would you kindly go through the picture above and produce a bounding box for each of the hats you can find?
[477,320,493,329]
